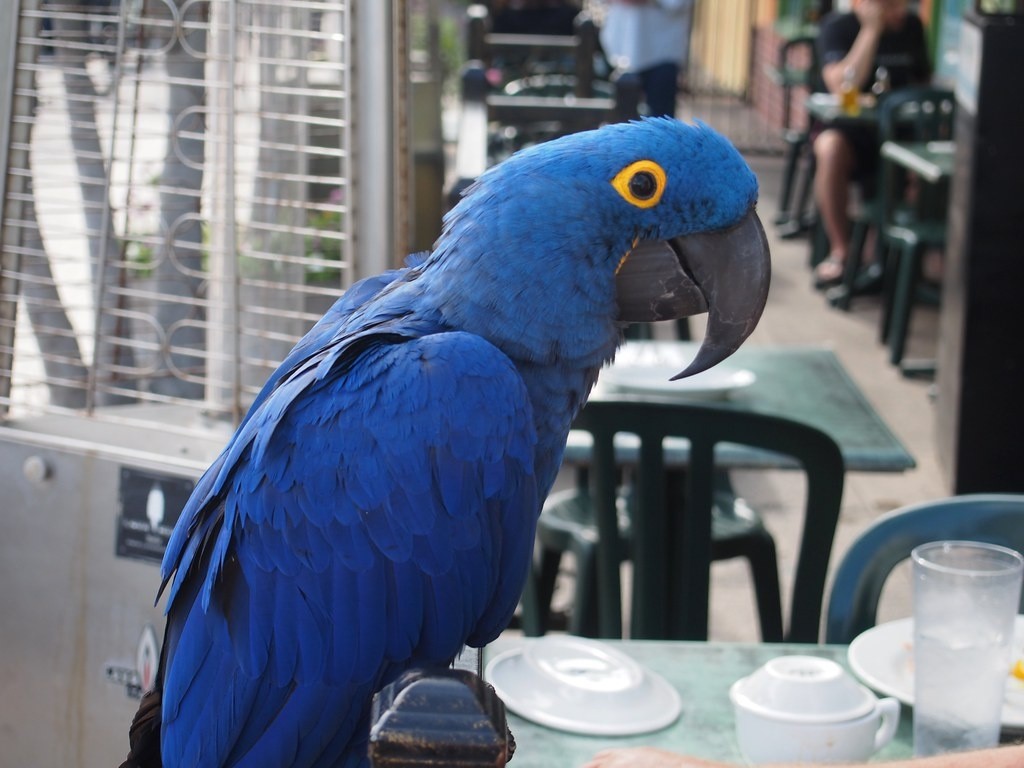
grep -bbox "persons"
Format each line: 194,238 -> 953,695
813,1 -> 949,288
594,0 -> 690,124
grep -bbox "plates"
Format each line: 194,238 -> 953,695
848,617 -> 1024,734
485,635 -> 683,736
594,341 -> 756,401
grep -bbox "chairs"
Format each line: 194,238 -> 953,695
774,38 -> 822,224
823,494 -> 1024,642
879,87 -> 953,364
537,317 -> 785,642
522,400 -> 846,643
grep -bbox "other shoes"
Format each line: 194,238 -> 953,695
814,259 -> 842,287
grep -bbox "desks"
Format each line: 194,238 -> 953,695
448,635 -> 915,768
802,88 -> 953,122
878,137 -> 955,194
564,336 -> 918,528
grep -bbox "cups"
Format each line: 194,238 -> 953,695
730,655 -> 900,762
912,540 -> 1024,759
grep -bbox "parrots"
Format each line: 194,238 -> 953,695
124,113 -> 770,768
